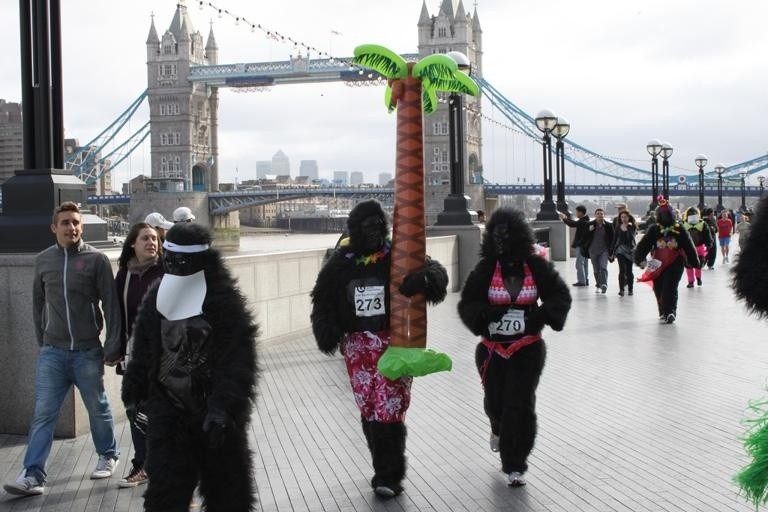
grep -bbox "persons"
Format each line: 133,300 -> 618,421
476,209 -> 487,223
113,206 -> 196,486
556,195 -> 754,325
4,201 -> 121,496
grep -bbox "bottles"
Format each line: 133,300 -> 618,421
615,203 -> 627,208
146,207 -> 195,230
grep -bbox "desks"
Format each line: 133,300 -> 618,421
693,154 -> 766,216
430,50 -> 480,227
658,142 -> 675,202
646,139 -> 663,217
548,117 -> 576,223
534,108 -> 564,222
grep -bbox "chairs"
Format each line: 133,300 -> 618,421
90,455 -> 119,478
4,477 -> 43,495
507,472 -> 526,486
619,290 -> 633,295
601,284 -> 606,293
659,313 -> 675,324
687,279 -> 702,288
491,433 -> 499,452
119,467 -> 149,487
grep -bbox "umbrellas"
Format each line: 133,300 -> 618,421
574,283 -> 585,286
